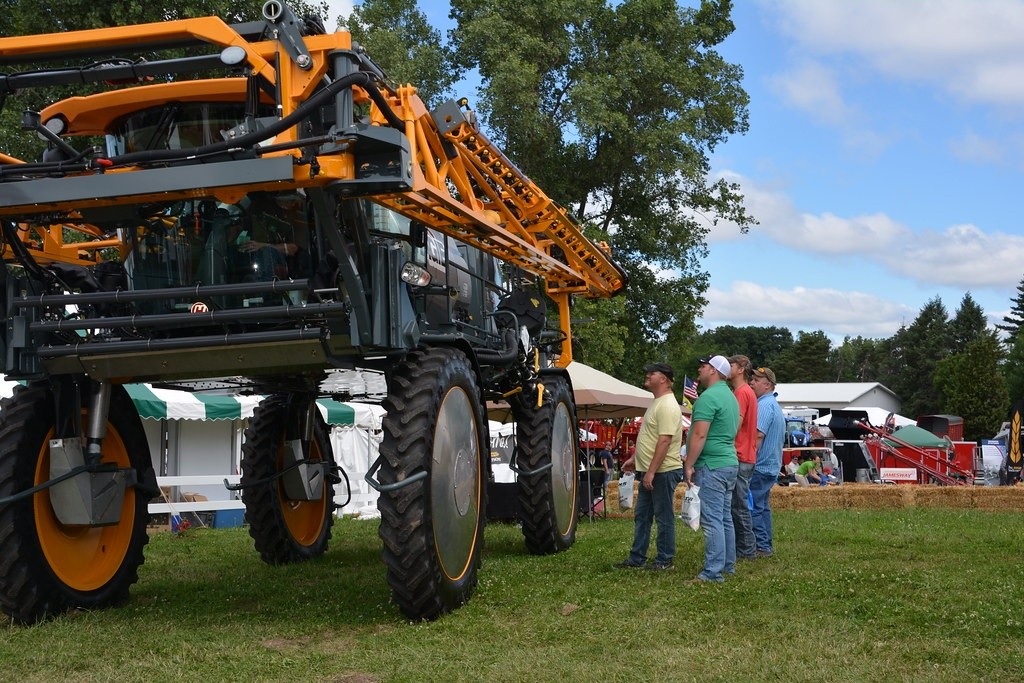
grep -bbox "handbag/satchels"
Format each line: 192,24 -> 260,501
681,482 -> 701,531
618,470 -> 635,513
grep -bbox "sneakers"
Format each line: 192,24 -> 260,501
646,562 -> 674,571
612,558 -> 646,570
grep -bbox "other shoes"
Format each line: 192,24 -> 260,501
685,572 -> 724,586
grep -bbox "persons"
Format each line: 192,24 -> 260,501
599,355 -> 837,583
230,241 -> 314,280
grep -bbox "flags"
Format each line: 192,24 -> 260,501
683,375 -> 698,399
682,395 -> 694,410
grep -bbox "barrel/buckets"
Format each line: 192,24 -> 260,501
215,510 -> 244,528
180,512 -> 215,528
855,469 -> 870,482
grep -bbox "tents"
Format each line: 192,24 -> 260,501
0,373 -> 390,521
879,425 -> 949,487
482,360 -> 655,521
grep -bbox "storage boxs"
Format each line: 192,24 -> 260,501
194,509 -> 247,530
181,492 -> 208,502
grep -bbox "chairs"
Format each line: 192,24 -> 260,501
577,469 -> 607,523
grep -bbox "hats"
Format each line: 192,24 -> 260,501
697,354 -> 731,377
752,367 -> 776,385
606,442 -> 612,446
642,362 -> 673,382
823,468 -> 831,474
725,354 -> 752,374
